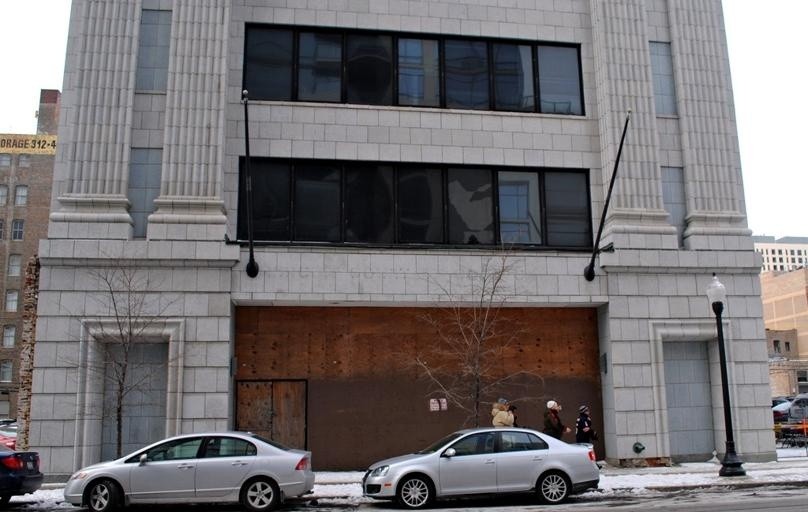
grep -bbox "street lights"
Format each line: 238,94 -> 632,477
705,271 -> 746,477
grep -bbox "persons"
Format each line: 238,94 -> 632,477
508,405 -> 519,427
576,404 -> 602,469
491,398 -> 515,448
542,400 -> 573,440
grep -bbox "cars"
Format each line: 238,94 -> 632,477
362,428 -> 600,508
0,418 -> 43,510
63,431 -> 315,511
772,393 -> 808,446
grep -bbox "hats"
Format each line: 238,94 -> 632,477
578,405 -> 589,414
498,397 -> 507,405
546,400 -> 557,409
